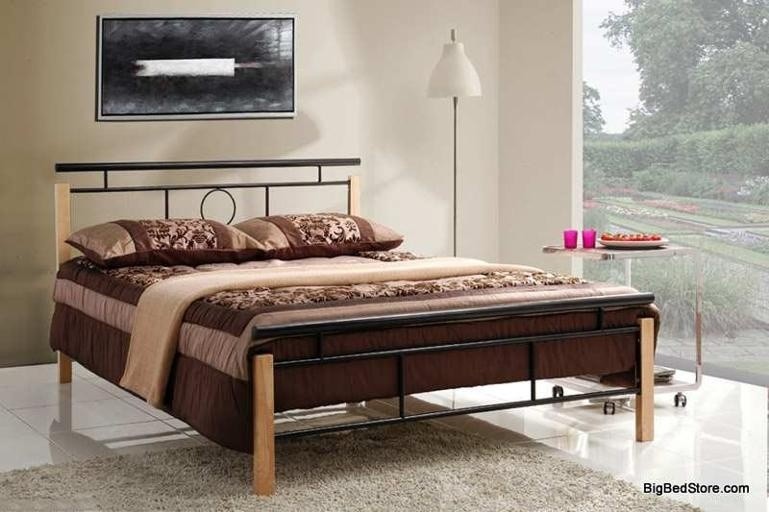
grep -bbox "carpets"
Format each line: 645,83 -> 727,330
1,420 -> 706,512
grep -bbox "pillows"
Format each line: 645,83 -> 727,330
64,219 -> 268,269
232,214 -> 404,260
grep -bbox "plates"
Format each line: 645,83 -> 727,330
596,234 -> 670,248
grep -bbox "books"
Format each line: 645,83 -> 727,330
575,363 -> 676,385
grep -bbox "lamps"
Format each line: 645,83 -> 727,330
426,28 -> 483,257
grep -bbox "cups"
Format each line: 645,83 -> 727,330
563,229 -> 596,249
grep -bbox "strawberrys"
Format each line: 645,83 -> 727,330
602,232 -> 661,240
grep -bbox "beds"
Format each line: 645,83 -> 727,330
53,158 -> 656,496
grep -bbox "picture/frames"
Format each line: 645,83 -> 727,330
95,14 -> 297,121
